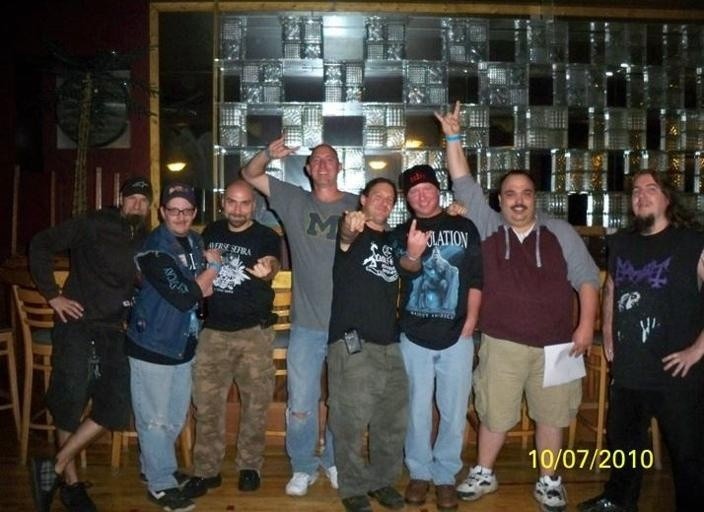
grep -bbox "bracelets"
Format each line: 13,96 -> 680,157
207,262 -> 220,272
402,252 -> 421,262
264,146 -> 272,161
445,134 -> 461,144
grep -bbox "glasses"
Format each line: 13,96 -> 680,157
164,209 -> 195,216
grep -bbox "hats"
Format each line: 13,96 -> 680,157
161,184 -> 197,209
122,178 -> 152,201
404,165 -> 440,197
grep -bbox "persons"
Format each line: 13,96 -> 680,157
433,98 -> 603,511
386,163 -> 483,512
324,178 -> 469,511
24,175 -> 155,511
184,179 -> 282,492
575,166 -> 703,512
121,182 -> 221,511
238,134 -> 390,496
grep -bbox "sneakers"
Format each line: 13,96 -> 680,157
27,454 -> 60,511
286,469 -> 318,496
534,475 -> 566,511
60,480 -> 98,512
139,473 -> 222,512
239,469 -> 260,490
325,466 -> 340,490
457,467 -> 497,501
343,480 -> 457,511
580,496 -> 633,510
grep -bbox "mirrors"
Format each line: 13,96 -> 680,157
148,1 -> 218,233
